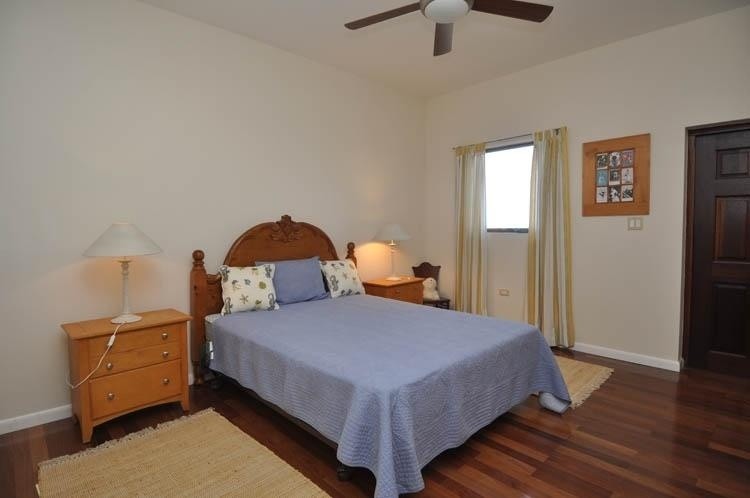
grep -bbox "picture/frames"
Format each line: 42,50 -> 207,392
581,133 -> 651,217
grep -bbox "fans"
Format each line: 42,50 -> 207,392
344,0 -> 554,56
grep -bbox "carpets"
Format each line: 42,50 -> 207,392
35,407 -> 334,498
531,353 -> 614,409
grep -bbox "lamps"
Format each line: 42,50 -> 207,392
373,223 -> 411,281
424,0 -> 469,24
82,223 -> 164,324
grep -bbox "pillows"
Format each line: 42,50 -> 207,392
318,258 -> 370,300
216,263 -> 279,317
255,256 -> 330,304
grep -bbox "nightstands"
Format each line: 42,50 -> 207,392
60,308 -> 193,442
362,276 -> 426,304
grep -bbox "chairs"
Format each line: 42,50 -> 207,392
412,262 -> 450,310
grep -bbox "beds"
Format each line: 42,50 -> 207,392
190,214 -> 540,498
